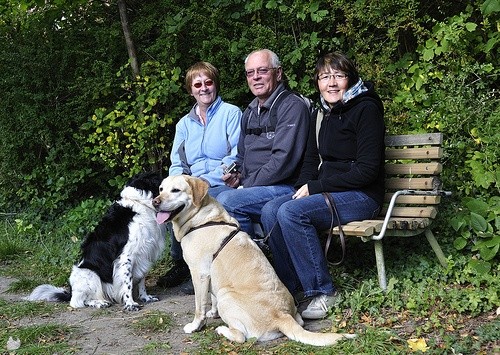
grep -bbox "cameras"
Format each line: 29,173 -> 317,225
223,162 -> 236,175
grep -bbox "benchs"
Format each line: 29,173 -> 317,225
323,133 -> 449,287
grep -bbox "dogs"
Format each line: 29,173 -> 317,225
27,158 -> 170,312
152,174 -> 358,346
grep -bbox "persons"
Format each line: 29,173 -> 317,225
156,61 -> 242,295
261,51 -> 386,319
207,48 -> 315,240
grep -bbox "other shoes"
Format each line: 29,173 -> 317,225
181,280 -> 195,295
157,264 -> 190,288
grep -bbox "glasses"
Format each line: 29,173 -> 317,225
317,70 -> 349,81
245,67 -> 277,77
191,80 -> 214,88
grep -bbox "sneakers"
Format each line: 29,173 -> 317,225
302,291 -> 338,318
